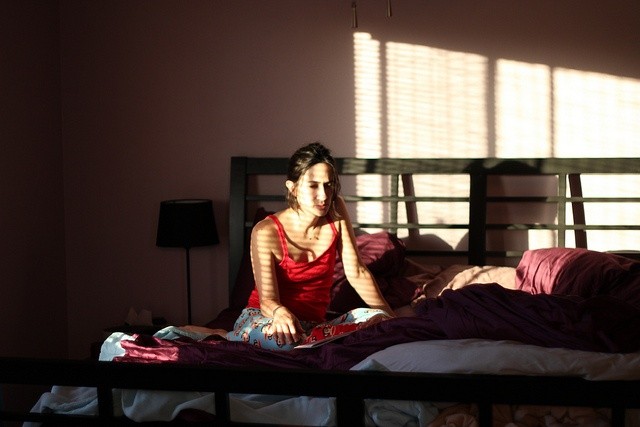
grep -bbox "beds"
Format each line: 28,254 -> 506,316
24,156 -> 639,427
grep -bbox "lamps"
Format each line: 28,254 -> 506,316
154,197 -> 221,325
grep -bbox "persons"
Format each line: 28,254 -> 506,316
177,143 -> 397,353
